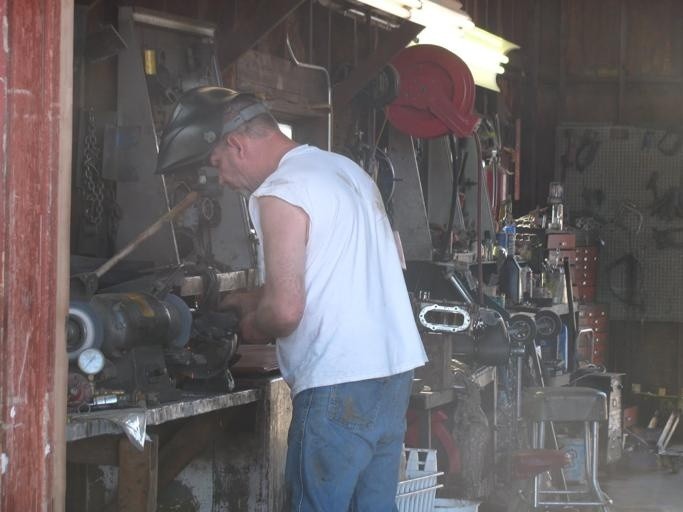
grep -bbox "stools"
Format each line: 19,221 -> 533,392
520,389 -> 613,512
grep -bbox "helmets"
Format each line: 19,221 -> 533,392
154,80 -> 257,176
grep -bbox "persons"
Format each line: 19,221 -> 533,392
202,90 -> 429,512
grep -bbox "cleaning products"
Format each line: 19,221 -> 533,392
496,227 -> 516,258
